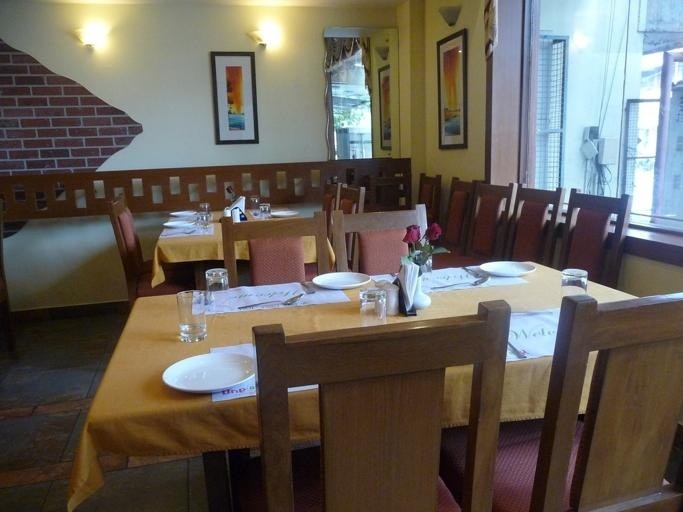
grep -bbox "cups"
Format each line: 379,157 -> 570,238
359,291 -> 388,332
204,269 -> 231,309
196,201 -> 210,233
176,288 -> 207,342
247,195 -> 272,219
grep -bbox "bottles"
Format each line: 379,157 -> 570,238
560,266 -> 588,294
375,279 -> 400,317
397,185 -> 407,210
226,185 -> 242,204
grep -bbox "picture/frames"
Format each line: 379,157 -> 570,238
209,50 -> 259,144
377,64 -> 392,151
435,27 -> 469,150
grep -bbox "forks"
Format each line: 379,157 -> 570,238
432,275 -> 491,291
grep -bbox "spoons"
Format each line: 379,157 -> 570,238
239,293 -> 303,311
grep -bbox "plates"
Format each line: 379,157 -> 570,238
311,271 -> 371,291
480,260 -> 538,276
270,207 -> 299,218
163,208 -> 196,228
160,354 -> 259,392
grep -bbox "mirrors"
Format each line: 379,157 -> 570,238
320,25 -> 402,162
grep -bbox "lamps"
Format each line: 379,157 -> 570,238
245,29 -> 278,49
71,28 -> 104,49
436,4 -> 462,26
